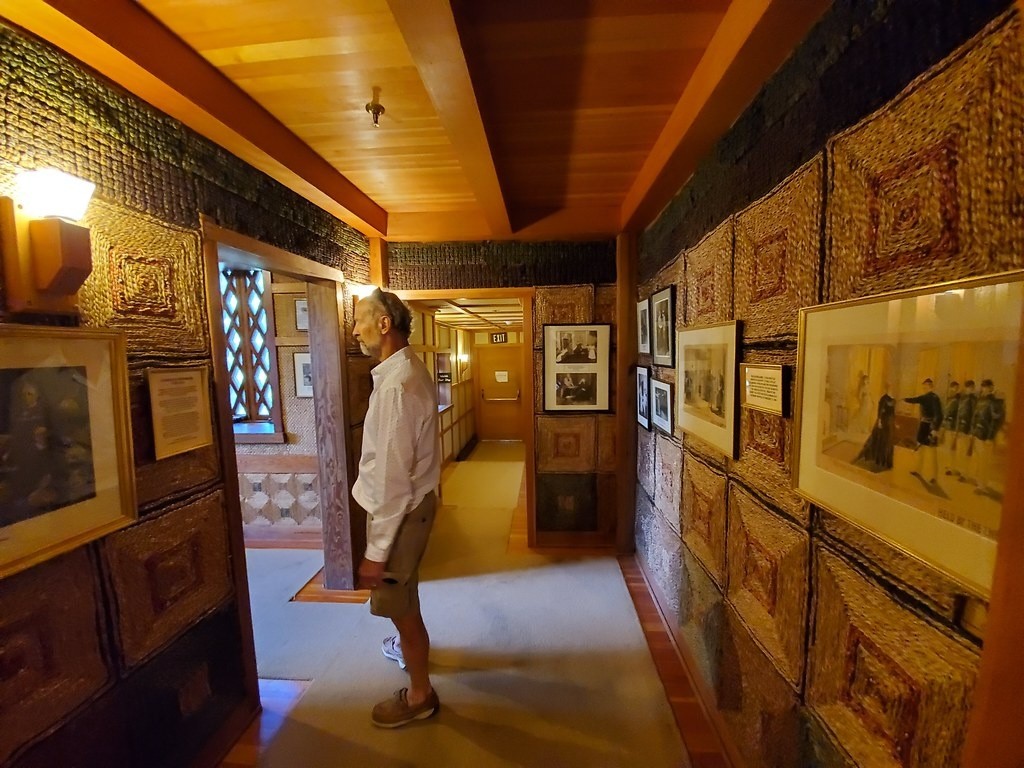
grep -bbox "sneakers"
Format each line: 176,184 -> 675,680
371,688 -> 440,729
381,636 -> 408,672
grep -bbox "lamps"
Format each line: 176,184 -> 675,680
22,167 -> 96,225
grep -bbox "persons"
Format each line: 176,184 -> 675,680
345,288 -> 446,727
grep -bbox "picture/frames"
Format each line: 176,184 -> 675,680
0,323 -> 140,579
144,366 -> 213,460
543,323 -> 610,414
293,352 -> 313,398
294,298 -> 308,331
651,284 -> 674,368
649,378 -> 674,437
739,363 -> 783,417
792,269 -> 1024,602
675,320 -> 739,458
636,298 -> 653,357
636,366 -> 651,431
